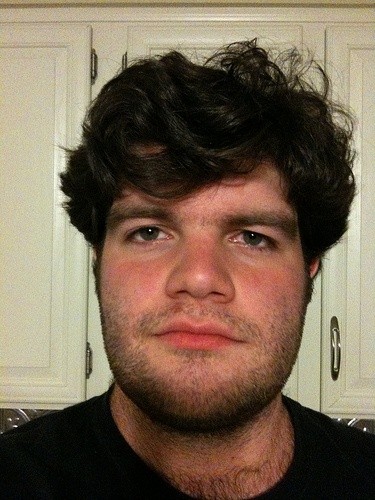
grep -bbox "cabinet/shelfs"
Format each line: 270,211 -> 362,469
0,0 -> 375,419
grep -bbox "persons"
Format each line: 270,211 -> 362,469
0,37 -> 375,500
